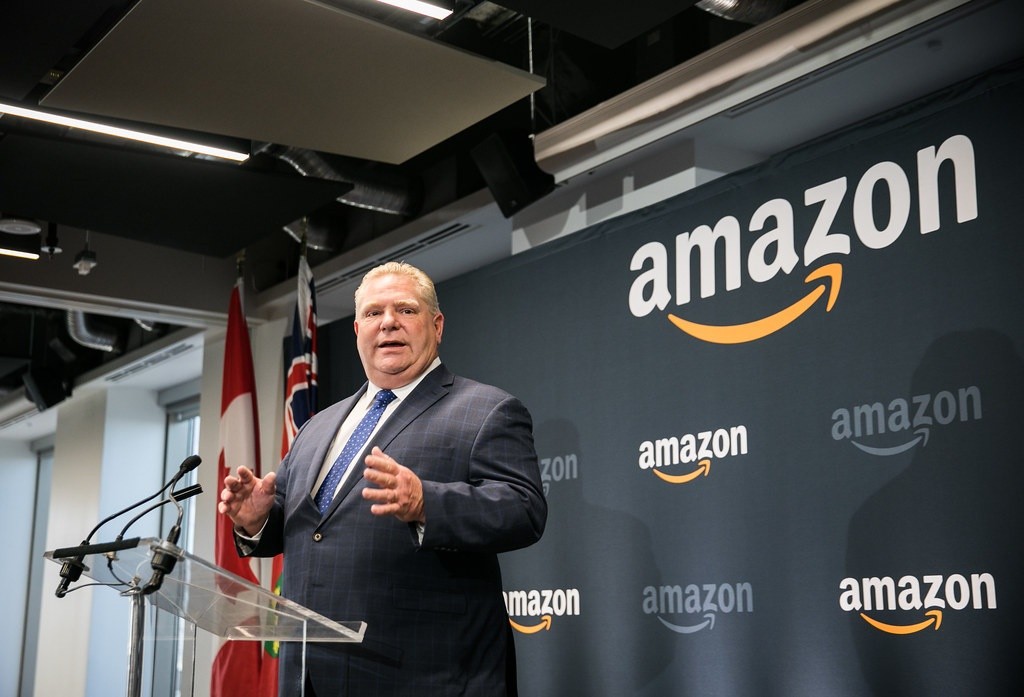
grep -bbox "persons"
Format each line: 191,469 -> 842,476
217,262 -> 548,697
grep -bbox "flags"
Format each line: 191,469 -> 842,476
260,258 -> 317,697
211,278 -> 260,697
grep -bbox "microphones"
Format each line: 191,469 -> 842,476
139,454 -> 202,596
55,456 -> 192,598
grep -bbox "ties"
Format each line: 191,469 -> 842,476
314,388 -> 400,515
